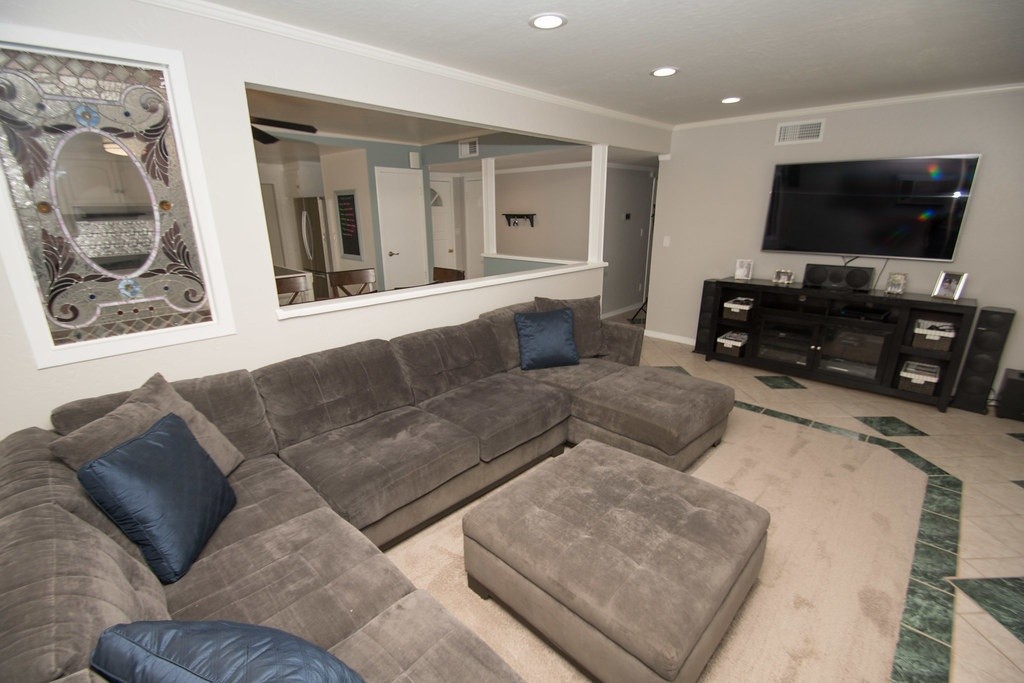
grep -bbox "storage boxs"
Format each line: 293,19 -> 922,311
716,330 -> 748,358
898,360 -> 941,397
723,297 -> 754,322
912,318 -> 956,352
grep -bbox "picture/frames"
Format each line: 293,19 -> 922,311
734,258 -> 753,280
930,270 -> 968,301
884,272 -> 909,294
0,23 -> 239,366
773,268 -> 796,285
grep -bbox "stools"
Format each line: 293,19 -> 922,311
461,438 -> 770,683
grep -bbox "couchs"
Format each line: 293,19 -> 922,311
0,293 -> 738,683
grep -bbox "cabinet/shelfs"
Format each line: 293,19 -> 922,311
693,276 -> 978,414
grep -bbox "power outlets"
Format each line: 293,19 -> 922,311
638,283 -> 641,292
640,227 -> 645,237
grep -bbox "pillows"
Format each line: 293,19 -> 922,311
514,307 -> 580,371
77,411 -> 238,586
46,370 -> 243,480
90,619 -> 367,683
535,295 -> 610,360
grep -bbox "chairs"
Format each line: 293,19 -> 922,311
275,267 -> 379,308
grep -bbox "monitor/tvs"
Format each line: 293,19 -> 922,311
759,154 -> 982,263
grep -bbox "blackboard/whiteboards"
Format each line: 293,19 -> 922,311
333,189 -> 363,261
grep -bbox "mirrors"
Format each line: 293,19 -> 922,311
48,126 -> 162,281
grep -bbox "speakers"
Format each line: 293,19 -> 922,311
802,263 -> 876,292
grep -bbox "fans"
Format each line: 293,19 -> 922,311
248,115 -> 320,144
